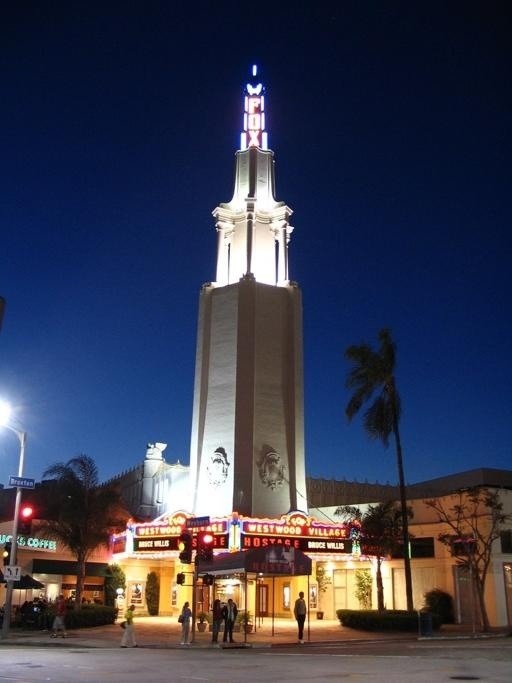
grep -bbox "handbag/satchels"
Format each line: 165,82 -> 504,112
120,621 -> 127,629
179,615 -> 185,623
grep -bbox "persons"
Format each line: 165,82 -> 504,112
294,591 -> 306,644
50,593 -> 68,637
180,602 -> 191,645
1,594 -> 103,612
212,599 -> 224,642
118,605 -> 137,648
221,599 -> 238,643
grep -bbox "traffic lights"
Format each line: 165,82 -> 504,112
17,502 -> 37,535
177,572 -> 185,583
197,530 -> 215,562
203,574 -> 214,585
179,530 -> 193,563
